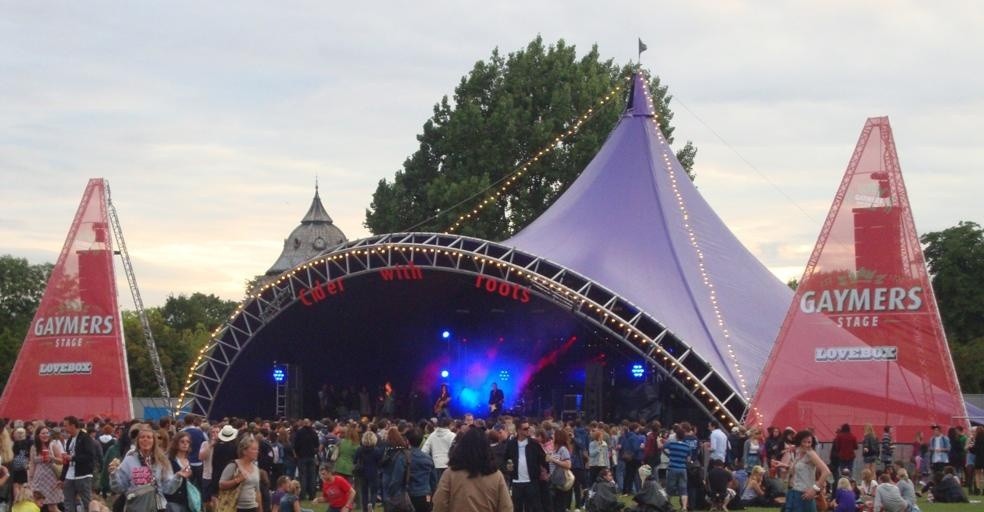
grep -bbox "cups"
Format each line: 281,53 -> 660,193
61,451 -> 69,465
40,449 -> 48,463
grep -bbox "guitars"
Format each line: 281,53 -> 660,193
489,399 -> 504,412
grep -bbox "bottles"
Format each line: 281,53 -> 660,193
506,459 -> 513,471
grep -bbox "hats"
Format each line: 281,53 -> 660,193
217,424 -> 238,442
639,465 -> 652,488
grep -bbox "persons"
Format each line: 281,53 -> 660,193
302,382 -> 373,419
488,382 -> 504,415
433,384 -> 451,417
380,382 -> 396,417
2,414 -> 982,512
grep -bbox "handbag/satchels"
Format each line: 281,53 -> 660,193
388,494 -> 412,511
123,485 -> 157,512
549,467 -> 574,491
186,478 -> 202,512
215,461 -> 244,511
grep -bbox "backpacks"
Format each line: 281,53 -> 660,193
324,436 -> 339,461
